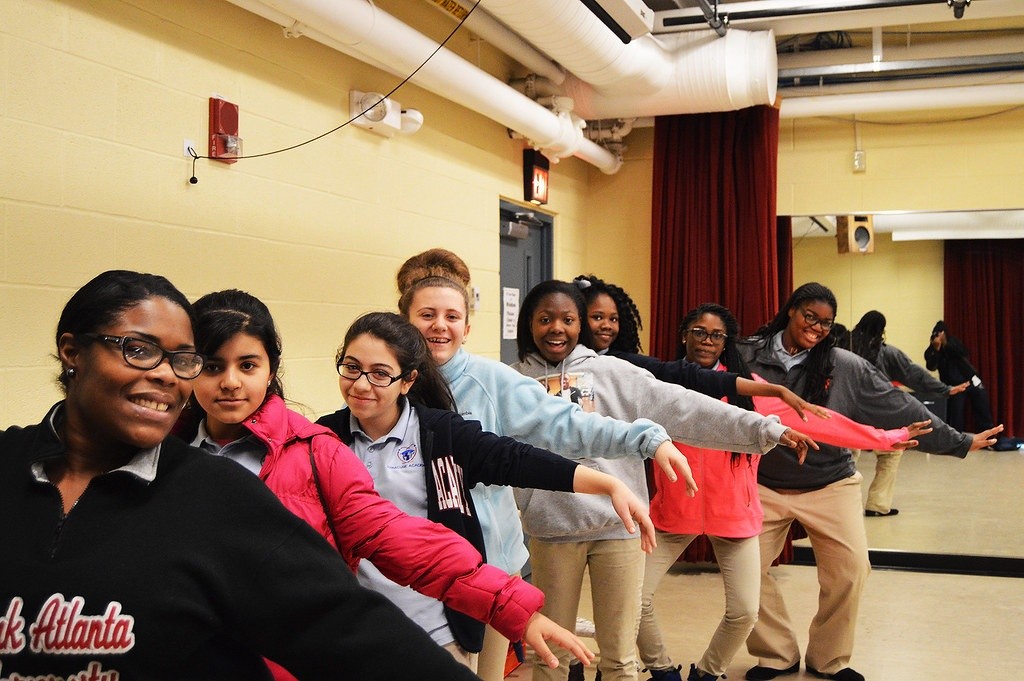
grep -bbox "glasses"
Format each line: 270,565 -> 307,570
798,305 -> 835,331
685,327 -> 728,345
74,331 -> 208,380
336,356 -> 410,388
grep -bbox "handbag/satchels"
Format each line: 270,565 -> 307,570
994,439 -> 1022,451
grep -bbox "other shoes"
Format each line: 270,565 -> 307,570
805,662 -> 864,681
746,662 -> 800,681
642,665 -> 682,681
688,663 -> 718,681
865,506 -> 898,517
568,662 -> 585,681
574,616 -> 597,638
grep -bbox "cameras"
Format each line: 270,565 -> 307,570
931,331 -> 939,340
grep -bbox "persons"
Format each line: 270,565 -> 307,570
504,280 -> 819,681
636,302 -> 932,681
734,285 -> 1005,681
397,248 -> 698,680
840,309 -> 972,516
568,274 -> 833,681
924,320 -> 989,451
0,269 -> 485,681
165,288 -> 597,681
312,312 -> 658,678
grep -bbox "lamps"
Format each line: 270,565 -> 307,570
349,89 -> 424,138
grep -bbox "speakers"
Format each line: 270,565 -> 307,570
836,214 -> 874,254
